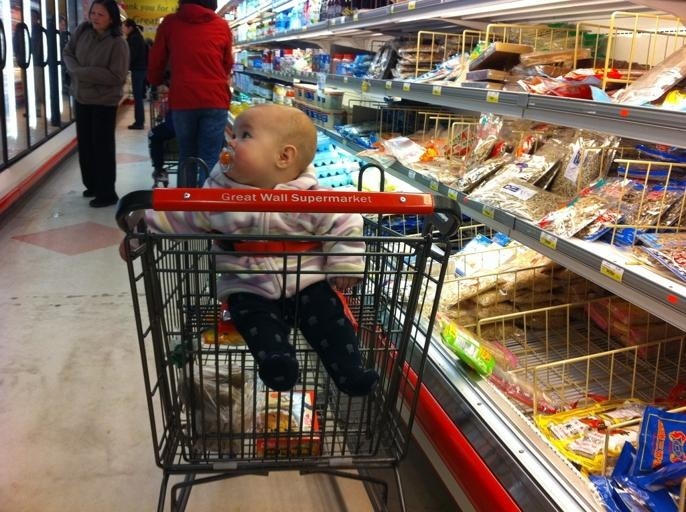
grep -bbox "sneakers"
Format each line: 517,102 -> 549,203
84,189 -> 97,197
89,191 -> 120,209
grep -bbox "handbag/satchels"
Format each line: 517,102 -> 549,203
63,25 -> 89,94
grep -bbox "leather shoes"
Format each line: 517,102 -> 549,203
128,124 -> 145,128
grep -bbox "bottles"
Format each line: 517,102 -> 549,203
253,79 -> 295,106
331,55 -> 354,78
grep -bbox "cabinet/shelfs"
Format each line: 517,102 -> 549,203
231,0 -> 685,512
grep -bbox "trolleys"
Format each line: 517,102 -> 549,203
118,165 -> 461,512
148,78 -> 203,188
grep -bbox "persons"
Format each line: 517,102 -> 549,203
146,0 -> 234,189
126,102 -> 379,400
61,0 -> 130,207
122,19 -> 147,130
